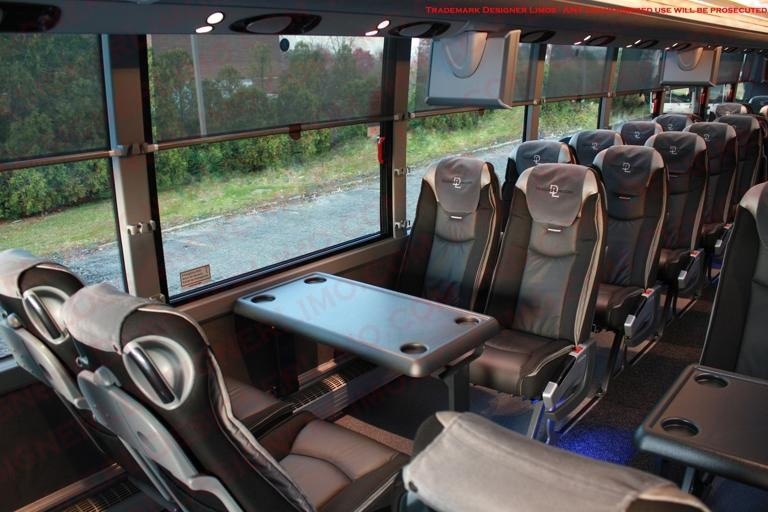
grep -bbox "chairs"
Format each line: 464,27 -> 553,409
62,283 -> 411,511
0,248 -> 295,493
398,411 -> 707,511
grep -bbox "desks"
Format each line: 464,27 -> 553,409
237,271 -> 499,412
635,362 -> 768,511
